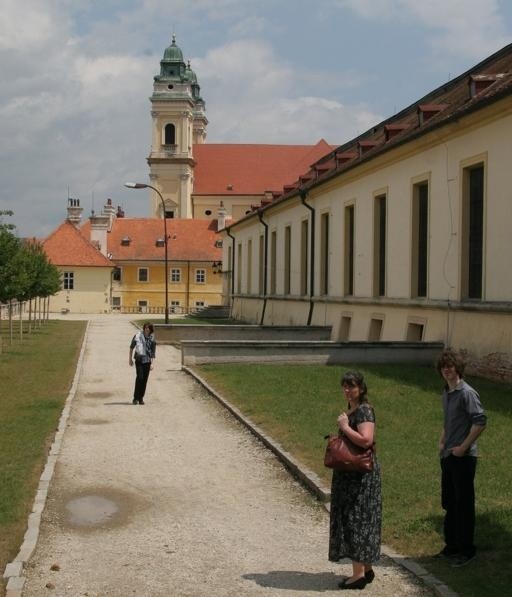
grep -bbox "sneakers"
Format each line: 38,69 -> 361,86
133,398 -> 144,406
431,545 -> 478,570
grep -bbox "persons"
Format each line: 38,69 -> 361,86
128,323 -> 157,405
328,368 -> 383,591
430,349 -> 488,568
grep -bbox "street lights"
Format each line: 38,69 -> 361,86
123,183 -> 169,324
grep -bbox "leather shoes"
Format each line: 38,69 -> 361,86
338,570 -> 376,591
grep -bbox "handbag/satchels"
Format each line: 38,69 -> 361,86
323,433 -> 375,473
